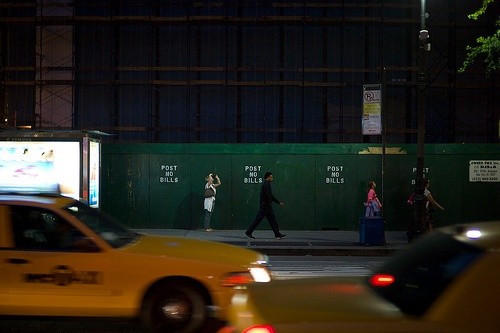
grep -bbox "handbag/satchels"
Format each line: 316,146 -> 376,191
203,188 -> 214,198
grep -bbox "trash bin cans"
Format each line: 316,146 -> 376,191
359,217 -> 384,245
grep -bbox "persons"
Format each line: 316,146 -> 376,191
244,172 -> 286,240
41,152 -> 48,161
367,180 -> 380,217
46,150 -> 55,159
23,148 -> 30,162
424,178 -> 445,233
203,172 -> 221,232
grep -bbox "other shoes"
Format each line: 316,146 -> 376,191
275,233 -> 286,239
201,228 -> 214,232
244,231 -> 255,240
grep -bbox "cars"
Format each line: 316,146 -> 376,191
218,221 -> 499,333
0,187 -> 271,333
